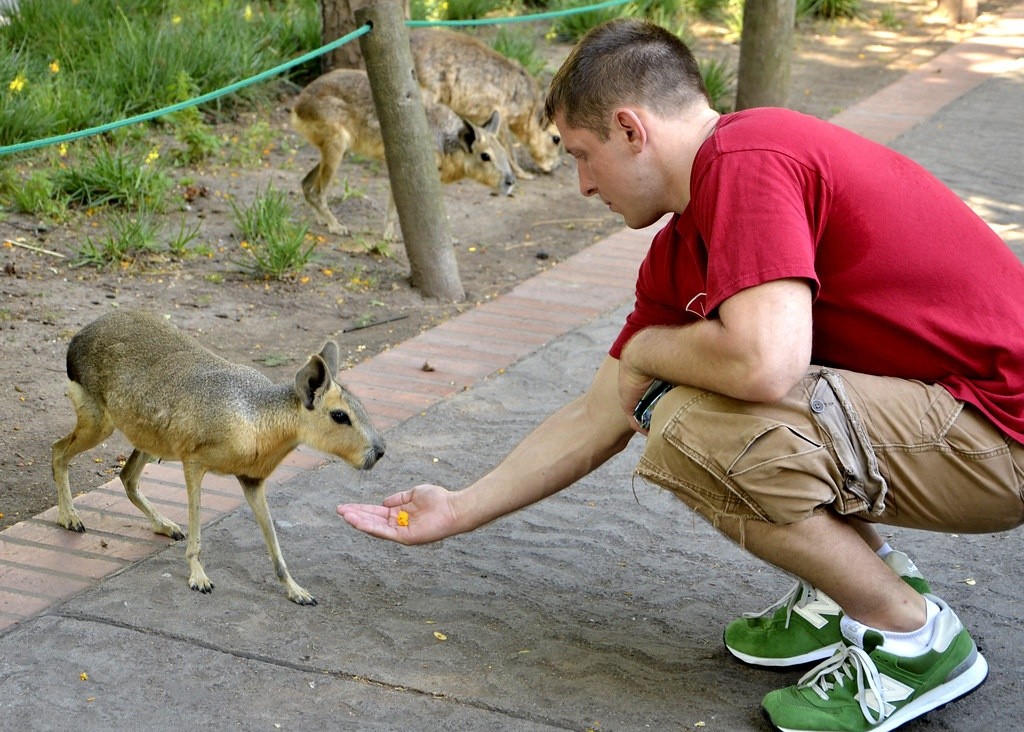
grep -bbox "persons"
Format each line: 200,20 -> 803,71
333,16 -> 1023,732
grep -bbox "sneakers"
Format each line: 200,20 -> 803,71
761,592 -> 989,732
723,549 -> 933,670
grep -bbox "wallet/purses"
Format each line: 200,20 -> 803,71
633,379 -> 674,429
